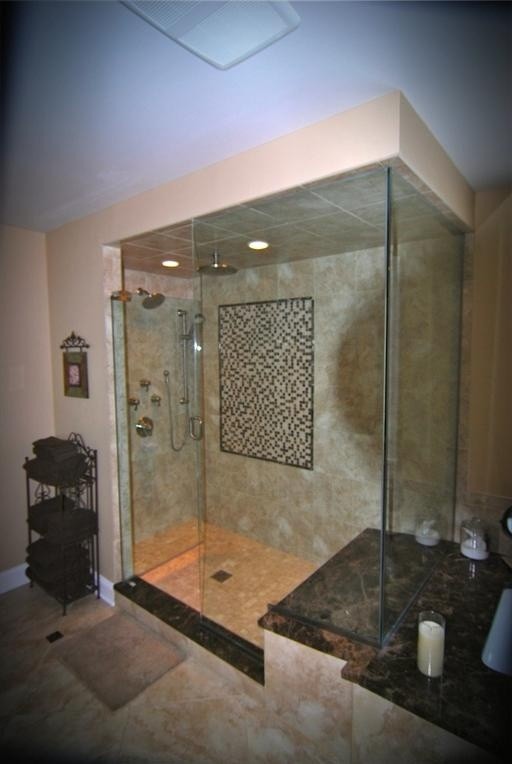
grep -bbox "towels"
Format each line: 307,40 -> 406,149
28,436 -> 96,598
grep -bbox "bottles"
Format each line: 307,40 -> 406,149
460,518 -> 491,561
415,513 -> 443,547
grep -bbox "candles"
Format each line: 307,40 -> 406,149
416,620 -> 446,677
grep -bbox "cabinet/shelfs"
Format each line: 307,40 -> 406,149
21,431 -> 101,615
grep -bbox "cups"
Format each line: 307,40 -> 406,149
418,611 -> 447,678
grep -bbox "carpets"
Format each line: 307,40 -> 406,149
47,609 -> 187,713
